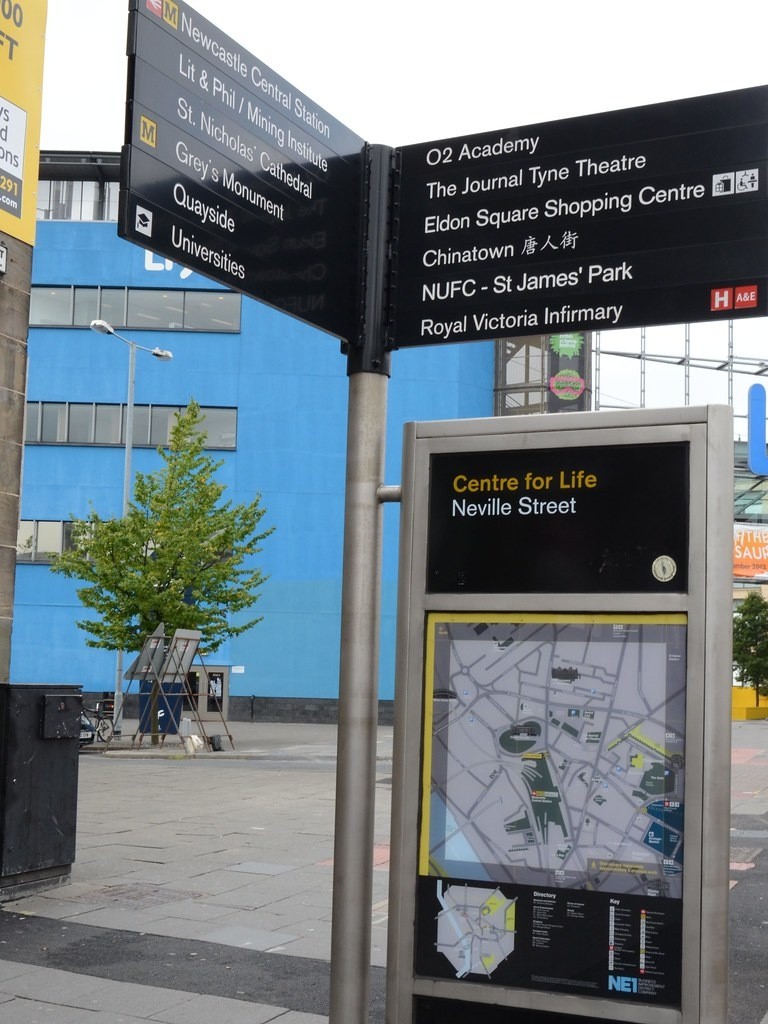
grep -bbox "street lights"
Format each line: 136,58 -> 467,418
88,318 -> 173,748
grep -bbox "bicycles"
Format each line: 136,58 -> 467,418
80,705 -> 114,743
